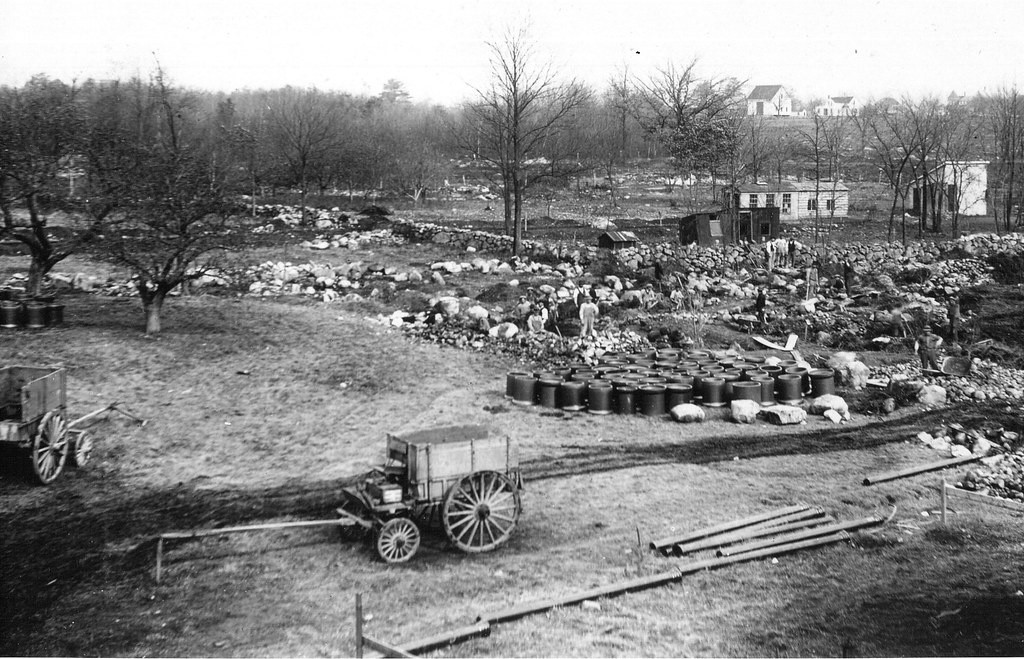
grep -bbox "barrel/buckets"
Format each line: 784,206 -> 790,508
0,289 -> 66,328
505,347 -> 836,416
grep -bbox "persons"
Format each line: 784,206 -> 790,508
805,261 -> 820,300
766,235 -> 795,271
638,284 -> 658,310
670,285 -> 683,312
891,304 -> 908,338
574,281 -> 599,338
914,326 -> 943,376
844,254 -> 855,297
947,296 -> 962,343
754,288 -> 766,322
516,284 -> 558,337
830,274 -> 845,299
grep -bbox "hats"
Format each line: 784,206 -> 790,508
646,284 -> 653,287
922,326 -> 933,331
532,307 -> 540,312
770,235 -> 776,239
790,236 -> 794,240
584,296 -> 593,299
579,281 -> 585,285
